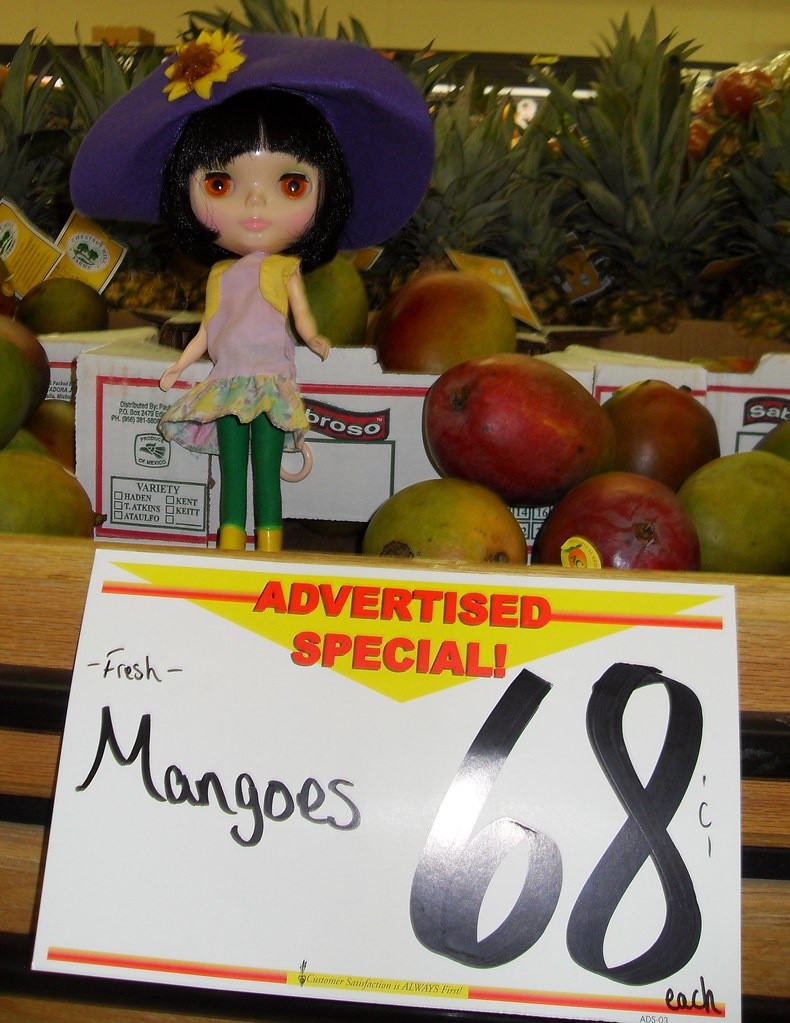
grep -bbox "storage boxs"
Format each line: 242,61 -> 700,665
564,346 -> 790,456
74,337 -> 594,565
38,328 -> 160,403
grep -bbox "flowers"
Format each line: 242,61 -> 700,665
162,30 -> 247,101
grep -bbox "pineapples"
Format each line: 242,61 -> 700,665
0,0 -> 790,357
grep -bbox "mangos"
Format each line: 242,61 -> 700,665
0,260 -> 110,538
295,258 -> 790,579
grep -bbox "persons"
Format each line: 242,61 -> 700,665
71,28 -> 435,554
